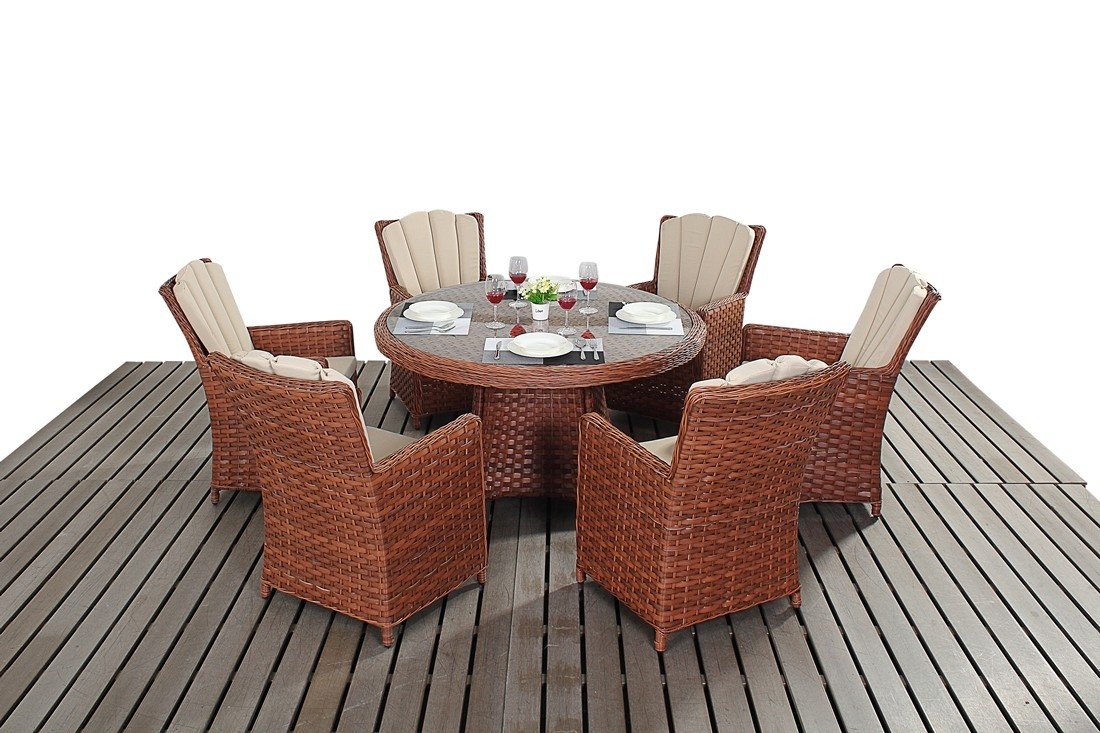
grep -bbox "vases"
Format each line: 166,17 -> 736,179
531,302 -> 549,320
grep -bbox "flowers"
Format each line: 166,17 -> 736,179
519,276 -> 559,303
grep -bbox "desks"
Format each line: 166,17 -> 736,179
373,280 -> 708,501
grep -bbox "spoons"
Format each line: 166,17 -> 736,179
405,321 -> 455,329
576,336 -> 588,360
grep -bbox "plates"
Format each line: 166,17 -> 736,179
402,301 -> 465,322
525,276 -> 577,293
615,302 -> 677,325
507,332 -> 574,358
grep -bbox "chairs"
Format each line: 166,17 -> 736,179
604,213 -> 766,423
575,354 -> 850,651
159,256 -> 363,503
207,349 -> 492,649
741,263 -> 942,516
374,209 -> 487,430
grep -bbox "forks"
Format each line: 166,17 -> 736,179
404,326 -> 455,333
590,338 -> 600,361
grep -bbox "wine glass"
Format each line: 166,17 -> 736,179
485,274 -> 505,329
556,279 -> 578,335
579,262 -> 598,315
509,307 -> 527,337
580,313 -> 596,339
509,256 -> 528,308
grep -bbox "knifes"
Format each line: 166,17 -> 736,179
619,326 -> 673,330
494,341 -> 502,359
